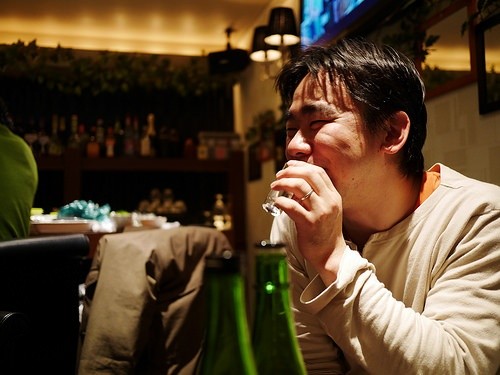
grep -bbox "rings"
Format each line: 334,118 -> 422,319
300,189 -> 314,202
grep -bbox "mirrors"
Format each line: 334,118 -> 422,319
417,0 -> 478,103
473,13 -> 500,115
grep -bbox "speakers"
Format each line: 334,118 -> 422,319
207,49 -> 251,75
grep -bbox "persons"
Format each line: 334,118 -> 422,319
270,36 -> 500,375
0,98 -> 38,242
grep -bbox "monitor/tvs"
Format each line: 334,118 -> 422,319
299,0 -> 375,56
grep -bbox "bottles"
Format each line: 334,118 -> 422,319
149,188 -> 174,214
214,192 -> 230,227
251,240 -> 309,375
198,256 -> 257,375
15,114 -> 160,163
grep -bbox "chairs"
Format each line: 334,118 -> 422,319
0,231 -> 91,375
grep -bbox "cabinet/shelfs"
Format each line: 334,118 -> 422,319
34,147 -> 245,249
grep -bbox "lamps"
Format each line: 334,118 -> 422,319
248,25 -> 280,79
264,7 -> 300,63
